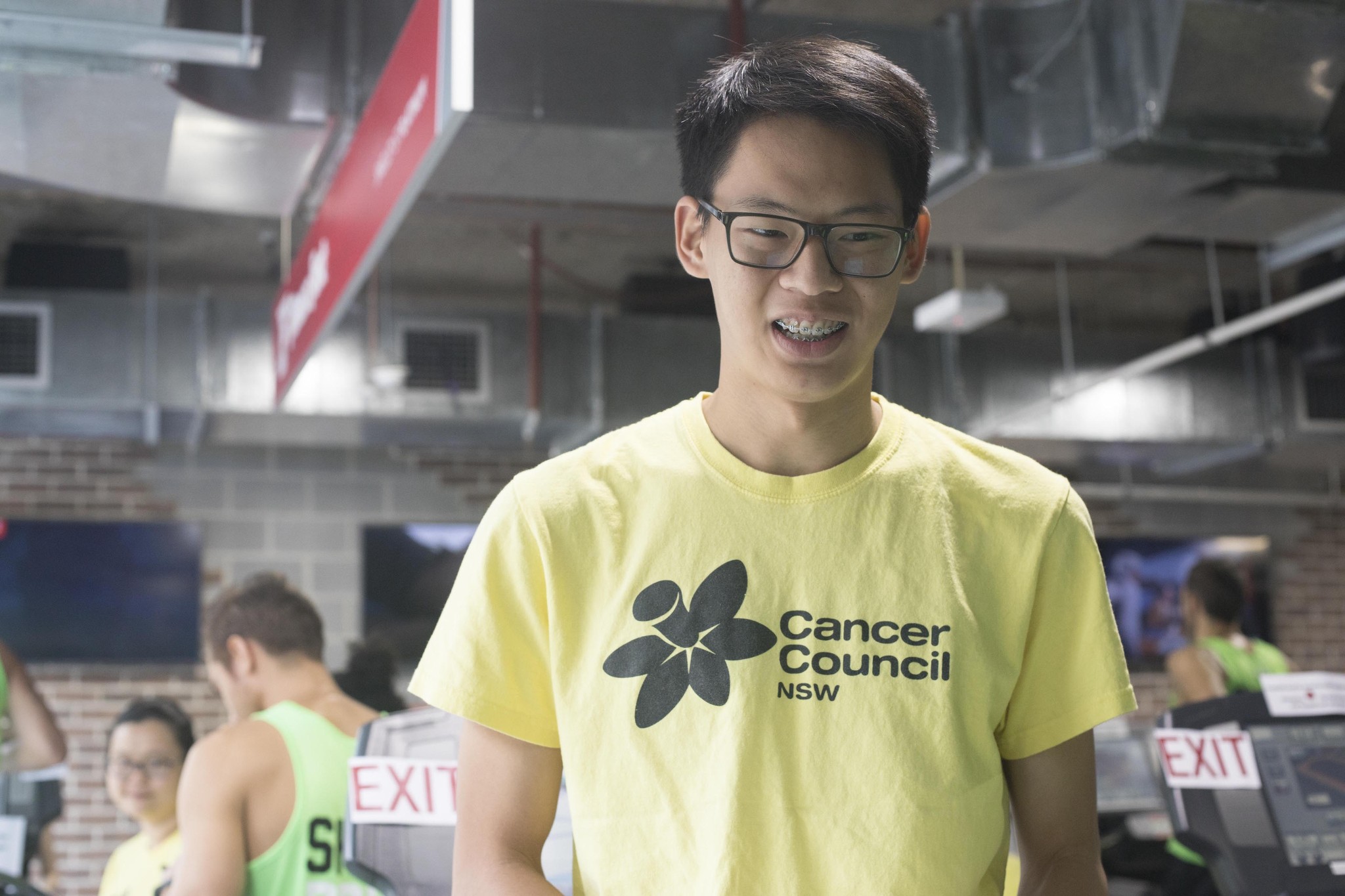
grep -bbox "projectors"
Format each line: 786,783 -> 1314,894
912,289 -> 1007,334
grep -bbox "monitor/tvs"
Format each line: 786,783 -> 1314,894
359,522 -> 484,672
1248,720 -> 1345,866
0,518 -> 205,667
1096,532 -> 1276,670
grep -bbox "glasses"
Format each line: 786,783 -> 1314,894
699,198 -> 918,278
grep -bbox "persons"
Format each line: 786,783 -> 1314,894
168,571 -> 387,896
409,39 -> 1141,896
1166,560 -> 1302,896
19,760 -> 68,893
99,695 -> 200,896
0,644 -> 67,778
